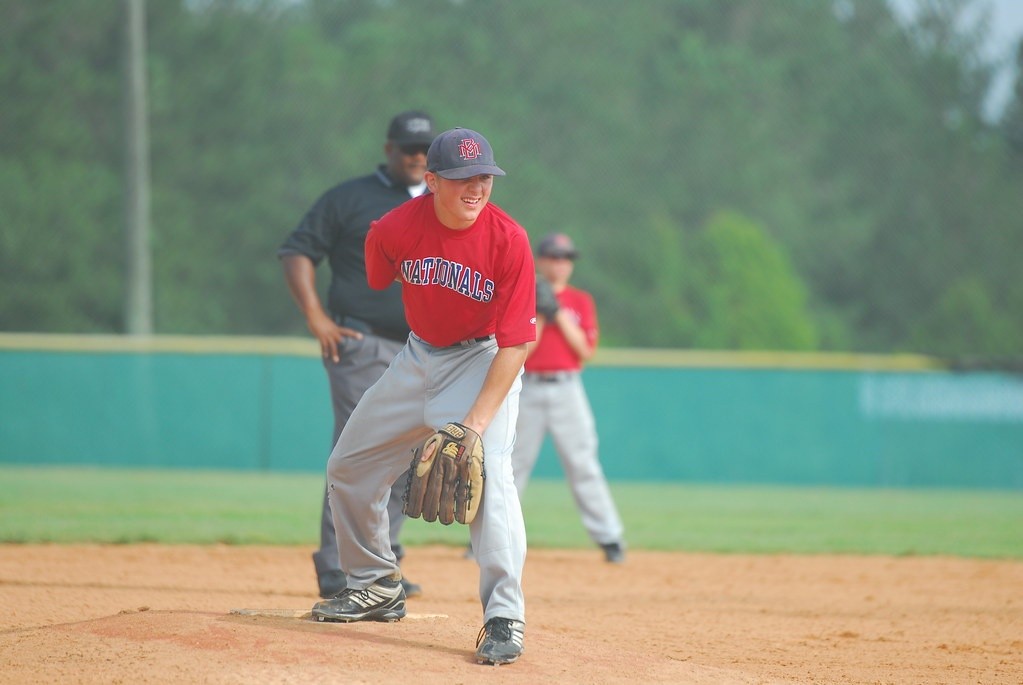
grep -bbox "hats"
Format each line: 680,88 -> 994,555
386,112 -> 436,145
425,126 -> 506,181
536,233 -> 579,261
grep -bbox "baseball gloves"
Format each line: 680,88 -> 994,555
401,420 -> 485,524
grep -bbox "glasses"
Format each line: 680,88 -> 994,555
398,145 -> 430,156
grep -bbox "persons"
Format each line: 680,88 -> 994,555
467,231 -> 626,565
310,125 -> 529,666
276,110 -> 434,600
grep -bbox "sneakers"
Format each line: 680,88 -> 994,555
399,576 -> 422,599
600,539 -> 625,564
463,543 -> 475,560
475,617 -> 526,666
311,576 -> 407,624
